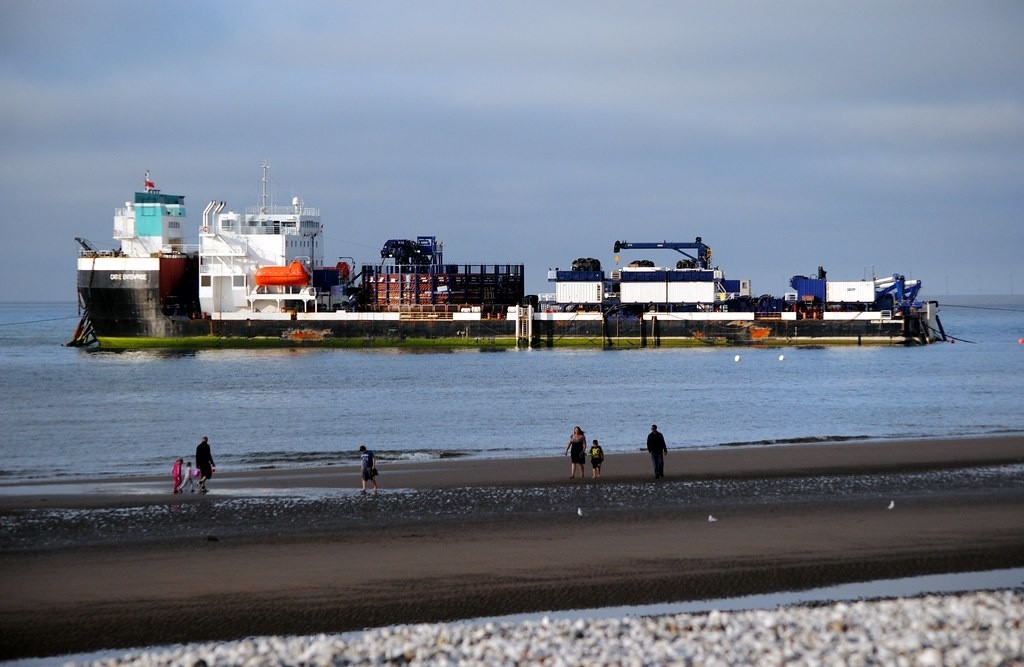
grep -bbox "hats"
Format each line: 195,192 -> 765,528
650,425 -> 657,429
176,458 -> 183,461
358,446 -> 366,452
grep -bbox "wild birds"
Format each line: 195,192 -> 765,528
708,514 -> 718,523
887,501 -> 896,510
577,507 -> 585,516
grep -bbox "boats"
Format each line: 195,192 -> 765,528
68,158 -> 977,348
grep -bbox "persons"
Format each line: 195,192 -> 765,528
196,436 -> 215,489
171,458 -> 183,494
565,427 -> 586,479
358,446 -> 378,492
176,461 -> 195,493
588,440 -> 604,479
647,425 -> 667,479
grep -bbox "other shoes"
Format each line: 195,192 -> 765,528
592,475 -> 596,479
196,481 -> 205,489
597,475 -> 601,478
174,490 -> 183,494
199,489 -> 209,493
579,477 -> 584,481
189,490 -> 195,493
176,488 -> 182,493
569,476 -> 574,479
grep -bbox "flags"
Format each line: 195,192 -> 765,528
146,182 -> 154,187
